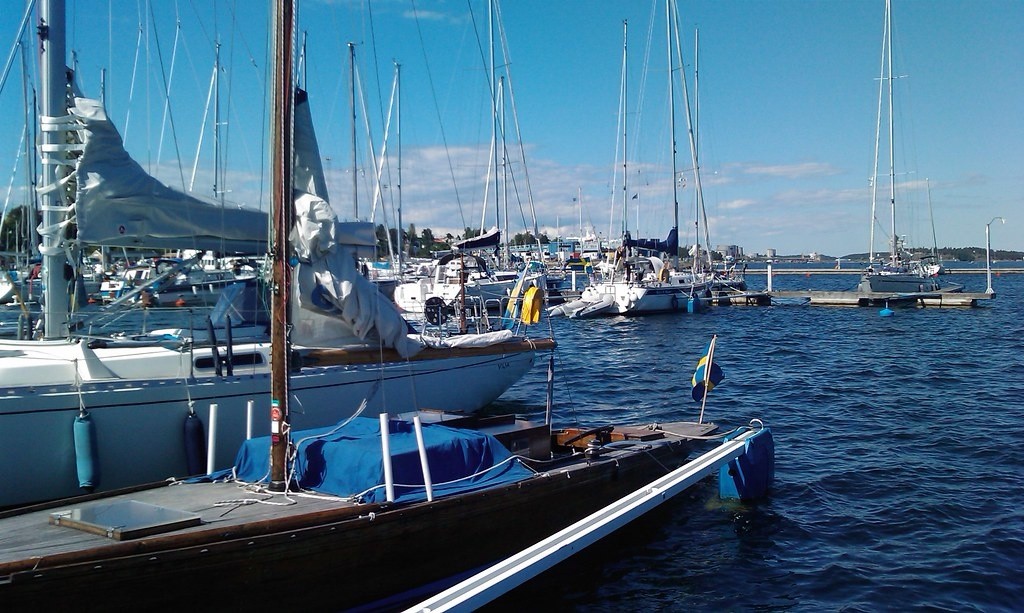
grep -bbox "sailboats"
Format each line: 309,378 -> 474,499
0,1 -> 768,613
868,1 -> 945,293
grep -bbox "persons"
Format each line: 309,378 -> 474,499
659,261 -> 669,284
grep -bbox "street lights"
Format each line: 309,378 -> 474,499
984,216 -> 1006,294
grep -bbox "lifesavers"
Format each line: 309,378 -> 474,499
521,286 -> 542,326
659,268 -> 670,283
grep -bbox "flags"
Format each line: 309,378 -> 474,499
500,259 -> 529,331
691,337 -> 725,403
